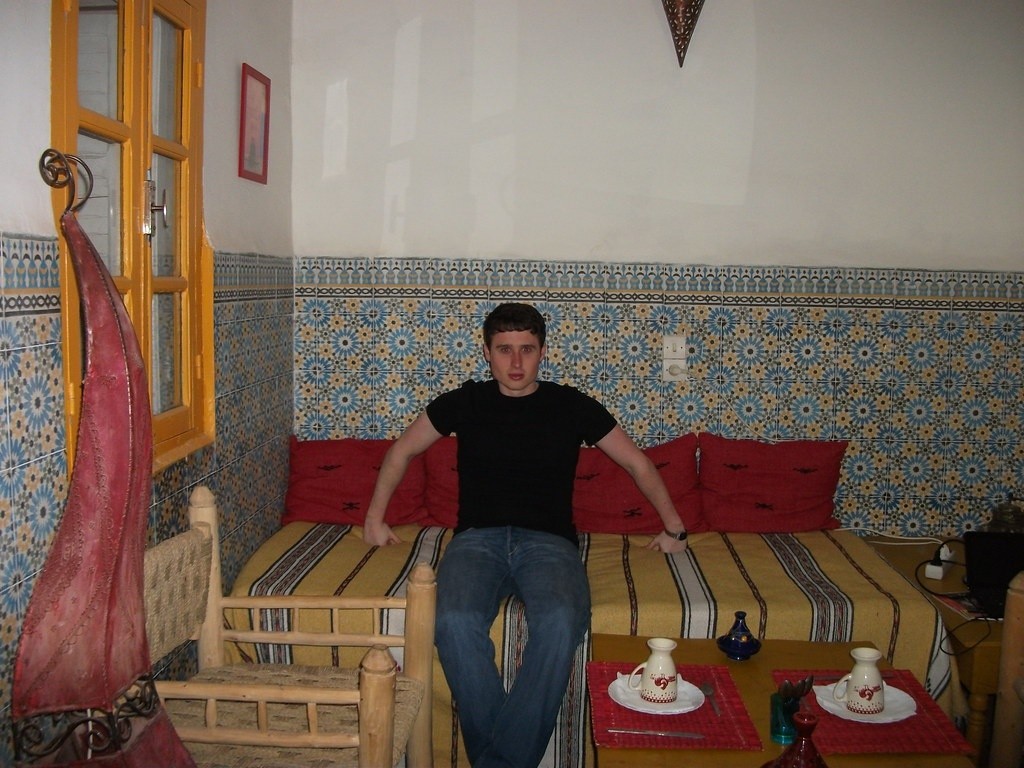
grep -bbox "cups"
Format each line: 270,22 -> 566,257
769,692 -> 800,746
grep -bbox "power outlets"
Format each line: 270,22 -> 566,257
663,359 -> 687,382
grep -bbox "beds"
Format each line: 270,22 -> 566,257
220,520 -> 968,768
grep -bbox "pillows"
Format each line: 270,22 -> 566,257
572,433 -> 710,535
417,436 -> 458,528
698,432 -> 847,531
281,435 -> 428,527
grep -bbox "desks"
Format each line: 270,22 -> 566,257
864,535 -> 1004,768
584,632 -> 975,768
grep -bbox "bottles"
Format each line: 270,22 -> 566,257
832,648 -> 885,715
629,638 -> 677,702
757,711 -> 831,768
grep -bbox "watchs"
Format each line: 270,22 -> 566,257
665,528 -> 688,541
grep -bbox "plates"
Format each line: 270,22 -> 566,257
816,682 -> 916,723
608,675 -> 706,715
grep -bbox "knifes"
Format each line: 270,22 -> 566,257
815,675 -> 896,680
607,728 -> 705,738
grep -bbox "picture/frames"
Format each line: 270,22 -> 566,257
238,62 -> 272,185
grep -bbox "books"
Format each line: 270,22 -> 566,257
932,594 -> 1003,621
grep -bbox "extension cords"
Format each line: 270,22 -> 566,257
926,550 -> 955,579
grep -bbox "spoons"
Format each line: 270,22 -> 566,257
700,682 -> 721,716
777,674 -> 813,706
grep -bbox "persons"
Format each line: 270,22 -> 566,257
363,303 -> 689,768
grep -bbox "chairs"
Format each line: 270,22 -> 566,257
143,485 -> 437,768
